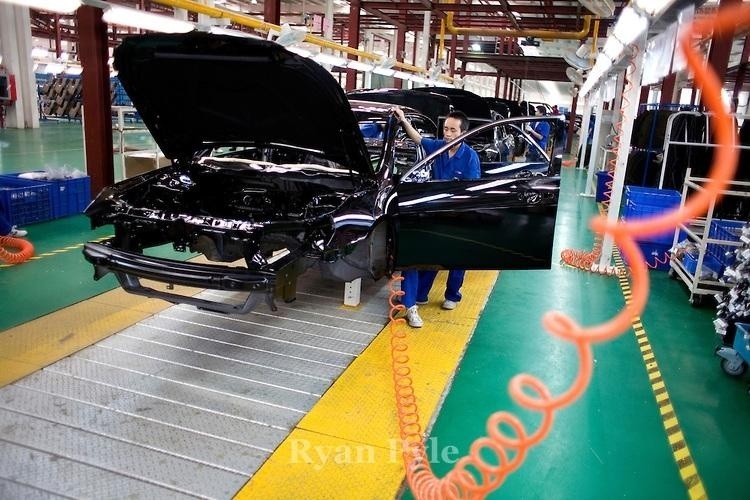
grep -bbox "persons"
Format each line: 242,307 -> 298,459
0,212 -> 28,237
361,105 -> 483,329
548,104 -> 558,117
524,104 -> 550,162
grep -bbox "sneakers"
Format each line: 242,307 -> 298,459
442,300 -> 457,309
406,307 -> 423,327
397,295 -> 428,304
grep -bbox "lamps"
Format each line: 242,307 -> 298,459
100,6 -> 456,93
561,1 -> 674,101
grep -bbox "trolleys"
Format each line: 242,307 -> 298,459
716,322 -> 750,376
668,168 -> 750,307
